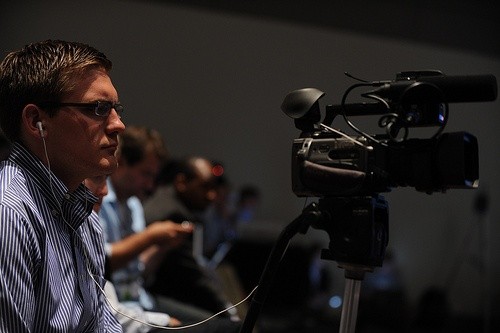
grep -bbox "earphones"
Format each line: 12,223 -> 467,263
36,121 -> 42,131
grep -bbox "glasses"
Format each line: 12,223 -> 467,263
57,99 -> 127,118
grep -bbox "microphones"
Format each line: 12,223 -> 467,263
374,75 -> 498,102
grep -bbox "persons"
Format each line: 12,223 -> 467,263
0,39 -> 127,333
80,124 -> 264,333
